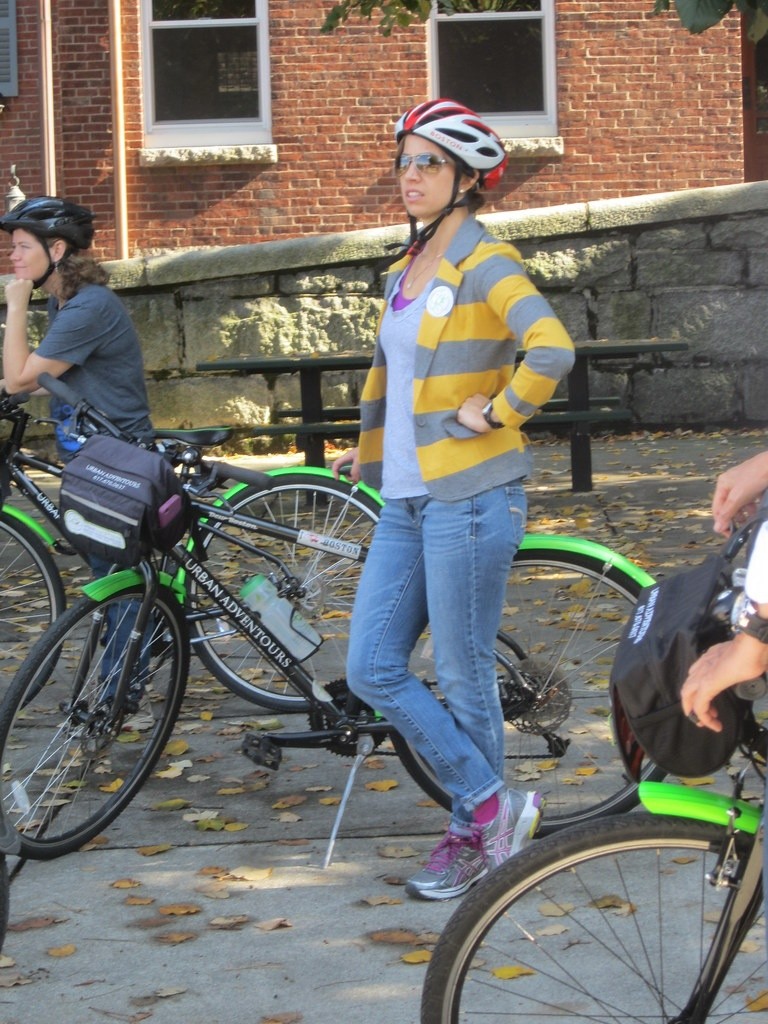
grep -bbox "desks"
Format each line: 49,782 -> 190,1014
194,335 -> 689,493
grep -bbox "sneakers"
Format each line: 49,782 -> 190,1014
404,817 -> 488,899
62,691 -> 154,739
470,788 -> 547,871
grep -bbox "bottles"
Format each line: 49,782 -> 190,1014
238,573 -> 323,660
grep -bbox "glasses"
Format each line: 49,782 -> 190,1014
396,153 -> 455,178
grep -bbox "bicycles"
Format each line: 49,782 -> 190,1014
1,366 -> 679,859
419,483 -> 768,1021
0,375 -> 388,721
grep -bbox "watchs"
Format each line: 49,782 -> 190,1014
481,402 -> 504,430
730,591 -> 768,645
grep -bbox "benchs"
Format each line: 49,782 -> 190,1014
254,392 -> 629,438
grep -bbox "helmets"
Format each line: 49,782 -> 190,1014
395,98 -> 508,190
55,419 -> 93,465
0,196 -> 97,248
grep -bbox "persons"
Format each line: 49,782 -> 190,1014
0,196 -> 156,738
332,99 -> 576,900
680,450 -> 768,734
